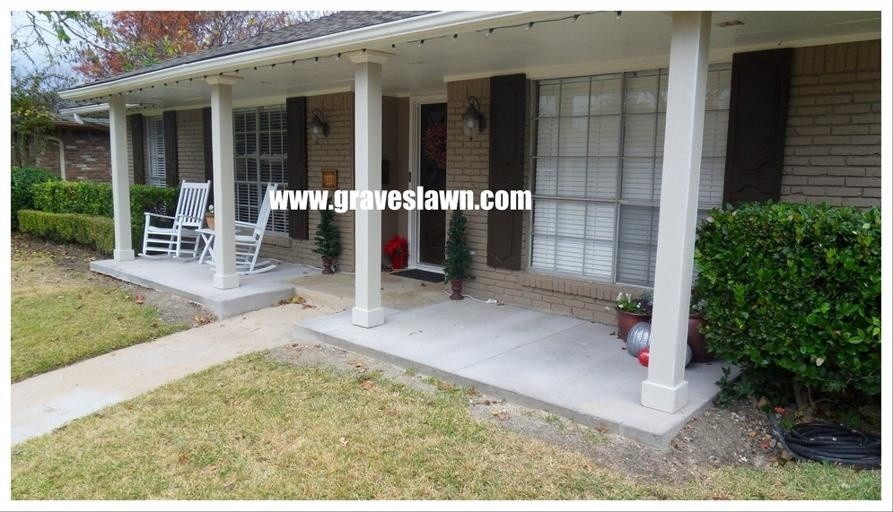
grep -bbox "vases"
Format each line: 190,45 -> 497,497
205,213 -> 214,229
688,314 -> 713,363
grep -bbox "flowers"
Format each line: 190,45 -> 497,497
206,204 -> 213,214
616,290 -> 646,314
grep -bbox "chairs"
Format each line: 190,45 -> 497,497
233,182 -> 278,277
136,178 -> 211,262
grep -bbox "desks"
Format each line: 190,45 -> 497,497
195,227 -> 242,263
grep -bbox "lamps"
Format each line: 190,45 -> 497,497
460,95 -> 487,144
308,107 -> 330,145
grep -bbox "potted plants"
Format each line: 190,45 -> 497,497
310,197 -> 343,275
441,200 -> 475,300
614,306 -> 650,342
